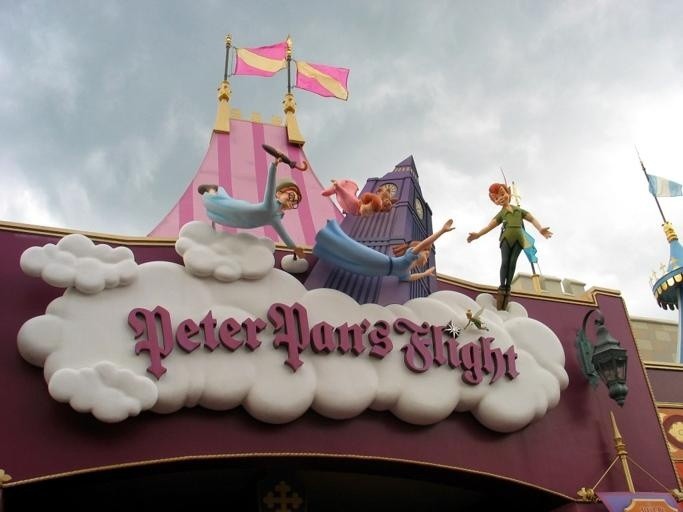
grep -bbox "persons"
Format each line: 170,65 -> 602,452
321,177 -> 393,217
466,183 -> 553,311
464,308 -> 490,332
198,156 -> 305,259
312,218 -> 456,282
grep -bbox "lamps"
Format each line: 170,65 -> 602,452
575,306 -> 631,412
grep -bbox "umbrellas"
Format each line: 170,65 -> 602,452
261,142 -> 308,171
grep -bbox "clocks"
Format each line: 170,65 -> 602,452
414,197 -> 424,221
377,183 -> 398,198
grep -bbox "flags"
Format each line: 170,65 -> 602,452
645,174 -> 683,197
291,57 -> 350,101
228,41 -> 288,78
